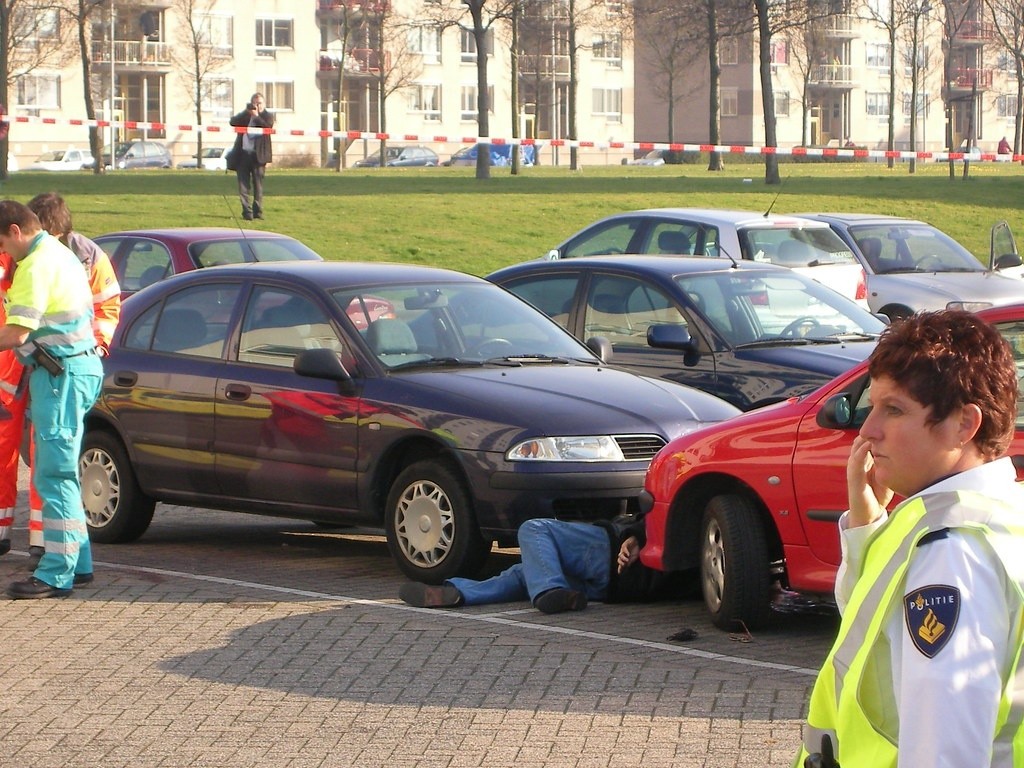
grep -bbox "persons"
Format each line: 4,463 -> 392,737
796,310 -> 1024,768
0,192 -> 121,599
399,510 -> 680,614
230,92 -> 273,222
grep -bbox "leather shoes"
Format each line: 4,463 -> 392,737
73,573 -> 94,587
6,576 -> 73,598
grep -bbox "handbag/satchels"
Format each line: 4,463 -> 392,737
225,152 -> 240,174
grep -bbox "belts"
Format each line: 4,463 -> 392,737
52,348 -> 94,359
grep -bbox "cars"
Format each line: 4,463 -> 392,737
80,141 -> 172,169
176,146 -> 232,171
18,147 -> 94,170
534,206 -> 870,335
630,149 -> 665,165
72,226 -> 395,376
342,254 -> 890,413
639,303 -> 1024,629
70,261 -> 752,584
355,145 -> 440,167
785,214 -> 1024,339
937,146 -> 985,162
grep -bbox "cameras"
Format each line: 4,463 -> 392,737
247,103 -> 257,110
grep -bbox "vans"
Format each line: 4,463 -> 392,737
441,144 -> 537,166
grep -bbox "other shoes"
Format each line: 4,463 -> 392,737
399,581 -> 464,608
257,217 -> 264,220
536,588 -> 587,613
0,539 -> 11,555
27,546 -> 45,570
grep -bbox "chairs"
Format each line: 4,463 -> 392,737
138,266 -> 170,290
776,239 -> 811,264
357,319 -> 434,367
859,237 -> 883,266
666,293 -> 725,339
591,280 -> 632,332
153,309 -> 208,349
657,231 -> 691,254
